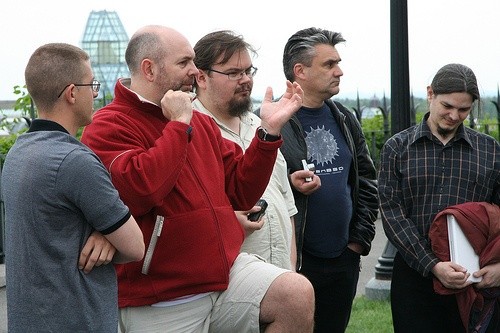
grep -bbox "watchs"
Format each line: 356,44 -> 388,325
258,126 -> 282,141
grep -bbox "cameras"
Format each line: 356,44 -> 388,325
301,159 -> 315,182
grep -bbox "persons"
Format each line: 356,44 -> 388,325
253,26 -> 380,332
378,63 -> 500,332
2,42 -> 145,333
187,29 -> 300,271
79,24 -> 316,332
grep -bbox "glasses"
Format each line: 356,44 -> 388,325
197,64 -> 258,82
58,80 -> 101,99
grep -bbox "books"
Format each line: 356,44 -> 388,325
448,213 -> 483,283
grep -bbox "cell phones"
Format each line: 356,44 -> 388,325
247,200 -> 268,222
